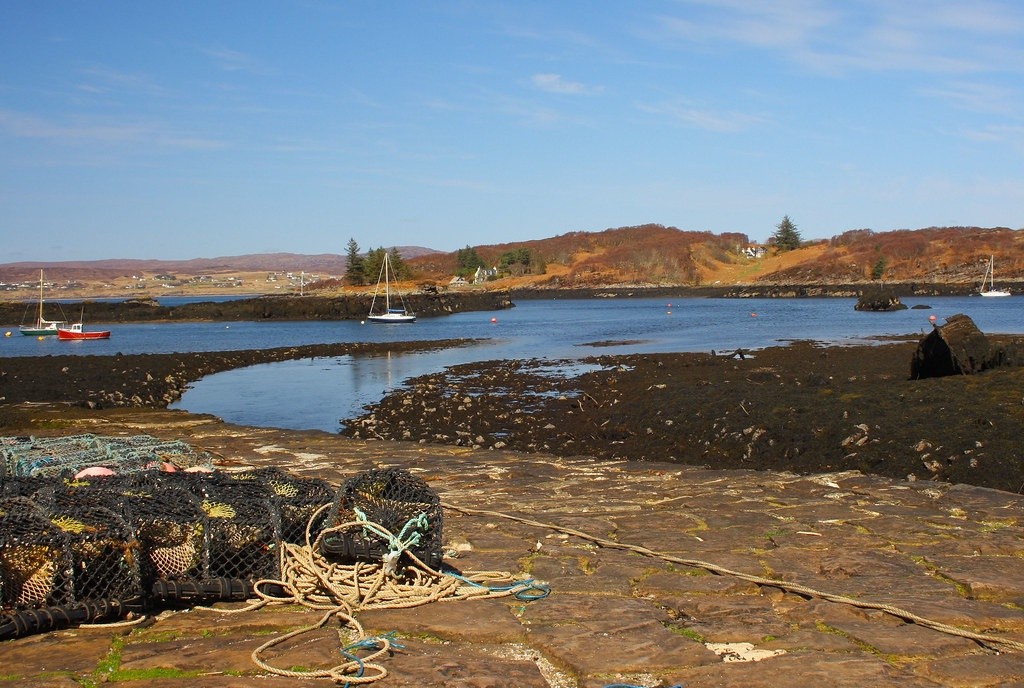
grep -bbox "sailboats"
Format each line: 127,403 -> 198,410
19,269 -> 69,335
979,255 -> 1011,297
366,253 -> 417,323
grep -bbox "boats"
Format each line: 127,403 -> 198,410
58,307 -> 111,341
